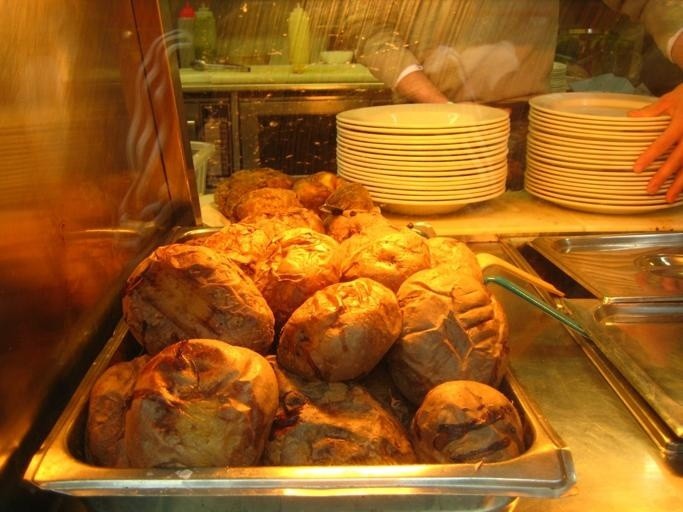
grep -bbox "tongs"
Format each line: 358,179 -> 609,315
189,53 -> 251,74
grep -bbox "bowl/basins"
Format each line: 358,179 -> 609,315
321,51 -> 355,67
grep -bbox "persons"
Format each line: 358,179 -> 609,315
343,1 -> 683,204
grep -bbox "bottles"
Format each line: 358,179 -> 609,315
176,1 -> 215,67
288,3 -> 310,66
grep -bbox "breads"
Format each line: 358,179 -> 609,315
88,167 -> 526,467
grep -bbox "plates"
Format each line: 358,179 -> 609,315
335,105 -> 511,215
523,91 -> 683,214
549,60 -> 567,90
